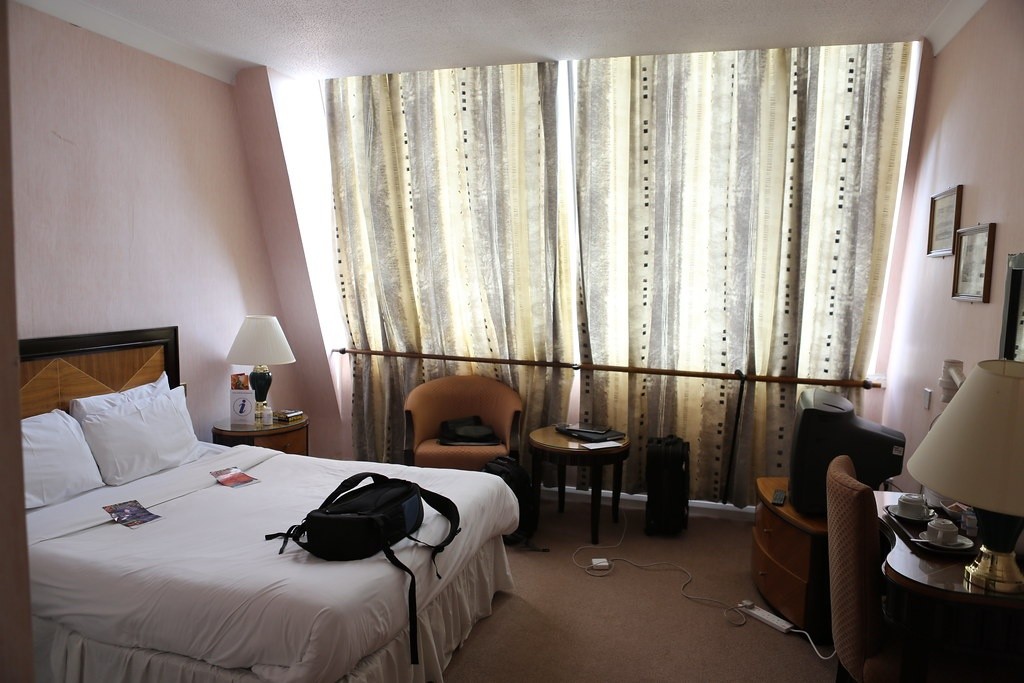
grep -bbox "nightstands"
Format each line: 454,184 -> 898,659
212,411 -> 309,454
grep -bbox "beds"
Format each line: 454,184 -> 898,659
18,326 -> 519,683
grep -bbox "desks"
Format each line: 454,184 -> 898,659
874,490 -> 1024,683
527,427 -> 632,545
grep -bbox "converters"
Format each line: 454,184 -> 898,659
592,559 -> 609,570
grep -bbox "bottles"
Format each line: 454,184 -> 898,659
263,407 -> 273,426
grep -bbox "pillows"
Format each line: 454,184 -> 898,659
69,369 -> 173,420
80,386 -> 203,484
22,409 -> 107,507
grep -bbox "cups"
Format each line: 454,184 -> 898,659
898,493 -> 929,519
926,518 -> 958,544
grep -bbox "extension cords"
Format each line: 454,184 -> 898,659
737,604 -> 795,633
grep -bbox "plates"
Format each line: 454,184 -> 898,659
919,531 -> 974,549
888,505 -> 938,521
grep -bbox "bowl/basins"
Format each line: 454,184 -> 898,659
940,500 -> 975,518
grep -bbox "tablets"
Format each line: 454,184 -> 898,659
565,422 -> 611,433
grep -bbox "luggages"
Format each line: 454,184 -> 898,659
645,435 -> 689,538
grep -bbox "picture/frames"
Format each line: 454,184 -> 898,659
926,184 -> 964,258
952,222 -> 996,304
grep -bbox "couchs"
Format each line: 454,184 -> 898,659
403,374 -> 527,480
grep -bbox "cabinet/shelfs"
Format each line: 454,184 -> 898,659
749,475 -> 839,632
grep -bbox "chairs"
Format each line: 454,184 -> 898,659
829,454 -> 967,683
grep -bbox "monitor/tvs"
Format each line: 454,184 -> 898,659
788,386 -> 906,521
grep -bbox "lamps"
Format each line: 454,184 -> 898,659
226,315 -> 296,418
905,357 -> 1024,593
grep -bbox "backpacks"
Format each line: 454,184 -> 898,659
265,470 -> 461,581
480,455 -> 541,546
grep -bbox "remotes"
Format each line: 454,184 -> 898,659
771,489 -> 786,506
